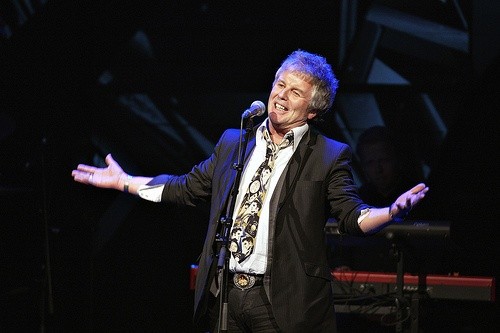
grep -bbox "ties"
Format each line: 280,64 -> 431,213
229,126 -> 295,261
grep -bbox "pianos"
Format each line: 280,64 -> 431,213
325,217 -> 452,240
191,264 -> 495,302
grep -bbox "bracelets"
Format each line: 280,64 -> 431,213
124,174 -> 133,193
388,202 -> 411,222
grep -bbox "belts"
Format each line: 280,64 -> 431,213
226,272 -> 264,289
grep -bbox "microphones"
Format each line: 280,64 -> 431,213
242,101 -> 266,118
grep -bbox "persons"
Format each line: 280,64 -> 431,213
323,125 -> 500,278
71,49 -> 429,333
